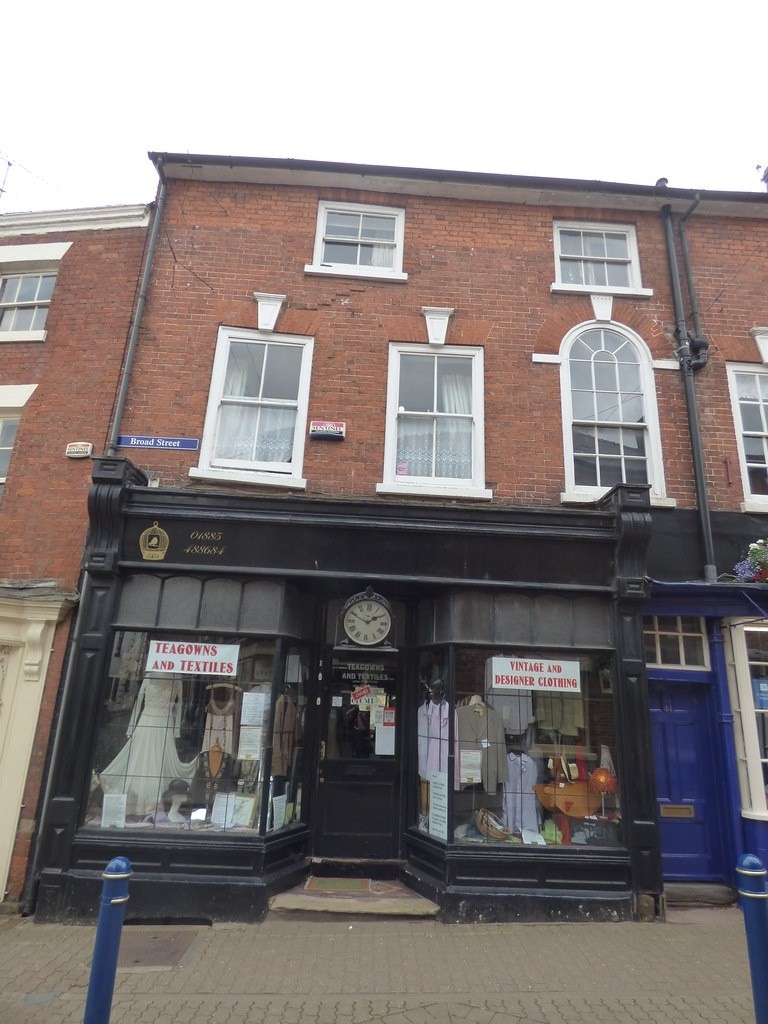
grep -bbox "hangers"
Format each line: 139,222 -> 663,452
425,688 -> 444,708
467,693 -> 485,712
206,681 -> 245,692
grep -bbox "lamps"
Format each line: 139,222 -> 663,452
589,767 -> 617,820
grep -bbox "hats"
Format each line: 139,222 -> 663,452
571,831 -> 587,845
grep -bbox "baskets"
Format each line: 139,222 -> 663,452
476,809 -> 512,840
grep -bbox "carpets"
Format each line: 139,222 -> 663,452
304,875 -> 371,893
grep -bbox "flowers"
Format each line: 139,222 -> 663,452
733,539 -> 768,583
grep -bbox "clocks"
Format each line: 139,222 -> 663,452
340,587 -> 395,646
